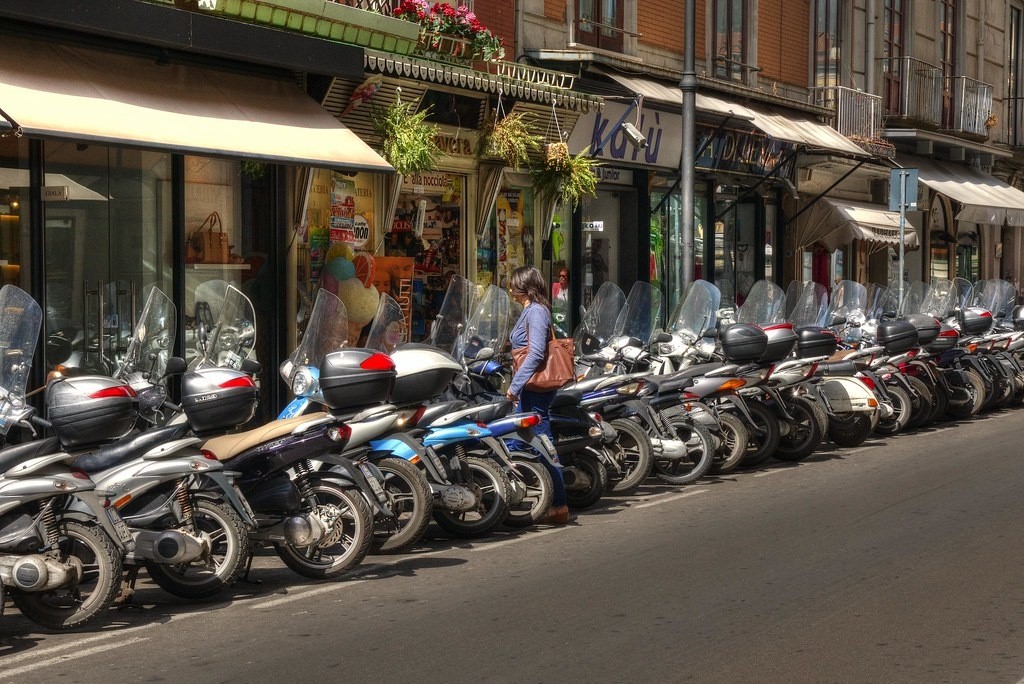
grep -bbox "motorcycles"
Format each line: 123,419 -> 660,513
75,284 -> 260,611
112,277 -> 1024,580
0,284 -> 141,631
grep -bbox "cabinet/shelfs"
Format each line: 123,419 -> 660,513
156,181 -> 255,343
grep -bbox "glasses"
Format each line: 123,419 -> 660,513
558,274 -> 566,278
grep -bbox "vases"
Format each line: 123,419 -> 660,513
416,30 -> 474,58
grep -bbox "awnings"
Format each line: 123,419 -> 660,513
1,46 -> 1024,247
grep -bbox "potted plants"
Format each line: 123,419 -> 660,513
475,87 -> 547,173
528,98 -> 609,212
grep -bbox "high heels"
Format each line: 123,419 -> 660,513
540,505 -> 570,527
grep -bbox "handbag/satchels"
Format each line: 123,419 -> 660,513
510,317 -> 574,389
185,211 -> 230,263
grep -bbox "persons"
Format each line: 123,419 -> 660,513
505,265 -> 569,524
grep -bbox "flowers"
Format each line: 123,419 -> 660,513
393,0 -> 505,61
